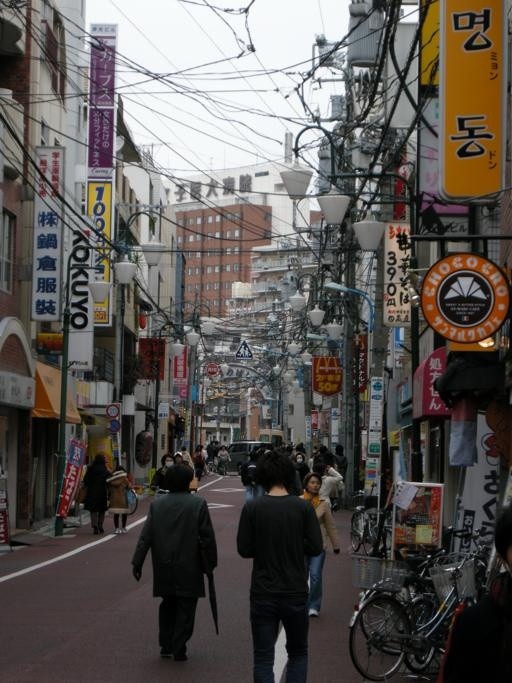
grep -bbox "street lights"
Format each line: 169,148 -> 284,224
280,123 -> 423,482
54,211 -> 165,538
288,272 -> 362,494
151,303 -> 216,494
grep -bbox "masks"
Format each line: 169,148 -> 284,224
297,459 -> 303,463
166,461 -> 174,468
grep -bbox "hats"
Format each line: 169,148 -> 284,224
173,451 -> 183,457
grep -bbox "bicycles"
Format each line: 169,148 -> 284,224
348,494 -> 494,682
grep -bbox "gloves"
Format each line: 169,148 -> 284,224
334,549 -> 340,554
133,565 -> 141,581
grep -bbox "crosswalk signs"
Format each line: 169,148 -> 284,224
235,341 -> 253,359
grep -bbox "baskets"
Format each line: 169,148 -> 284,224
350,554 -> 409,592
428,559 -> 476,601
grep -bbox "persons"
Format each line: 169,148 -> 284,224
297,473 -> 340,617
151,436 -> 231,494
437,506 -> 512,683
240,435 -> 348,512
236,452 -> 323,682
82,455 -> 132,535
131,462 -> 218,660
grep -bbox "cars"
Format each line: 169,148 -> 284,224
226,442 -> 275,475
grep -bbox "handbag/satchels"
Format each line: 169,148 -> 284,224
127,488 -> 134,504
78,484 -> 87,503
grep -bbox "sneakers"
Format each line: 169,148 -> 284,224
93,527 -> 128,534
308,608 -> 319,617
160,647 -> 173,656
332,500 -> 338,510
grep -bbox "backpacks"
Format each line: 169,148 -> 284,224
247,462 -> 257,481
